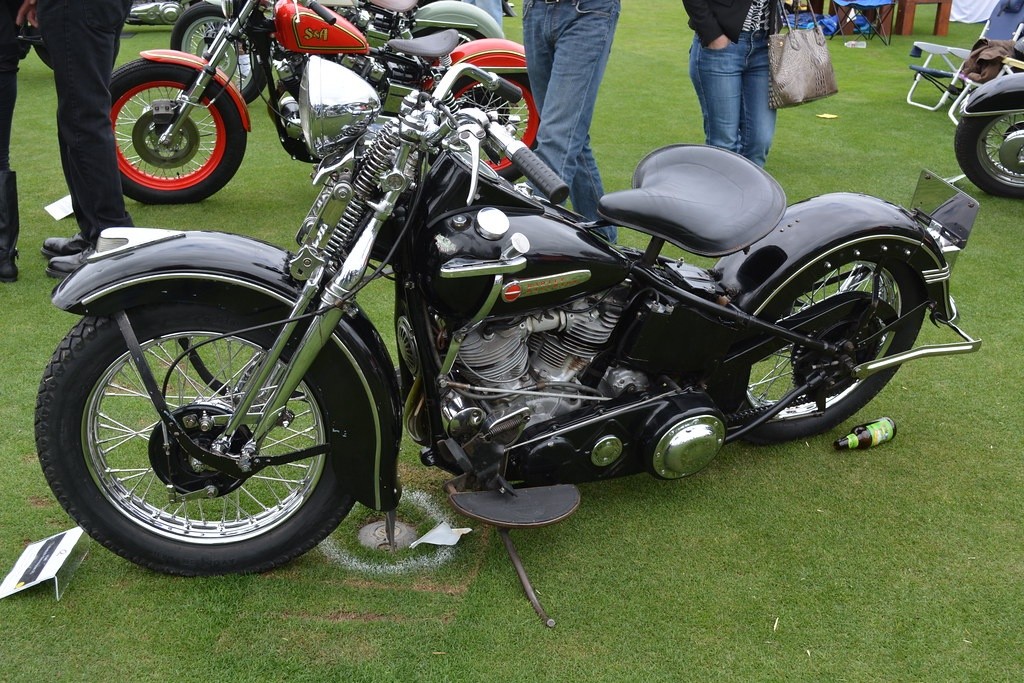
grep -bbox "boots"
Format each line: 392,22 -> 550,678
1,172 -> 19,283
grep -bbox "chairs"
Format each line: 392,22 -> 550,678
906,0 -> 1024,111
829,0 -> 896,49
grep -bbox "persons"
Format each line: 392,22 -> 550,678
459,0 -> 503,33
521,0 -> 620,247
0,0 -> 28,282
681,0 -> 785,171
16,0 -> 137,279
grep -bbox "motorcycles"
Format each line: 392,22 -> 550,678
954,72 -> 1024,201
33,61 -> 983,579
170,0 -> 505,106
108,0 -> 541,206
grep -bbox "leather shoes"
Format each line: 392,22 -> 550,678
46,245 -> 99,278
41,232 -> 92,259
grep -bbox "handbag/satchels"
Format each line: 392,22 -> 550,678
764,0 -> 839,108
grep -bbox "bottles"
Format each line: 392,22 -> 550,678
834,414 -> 897,451
